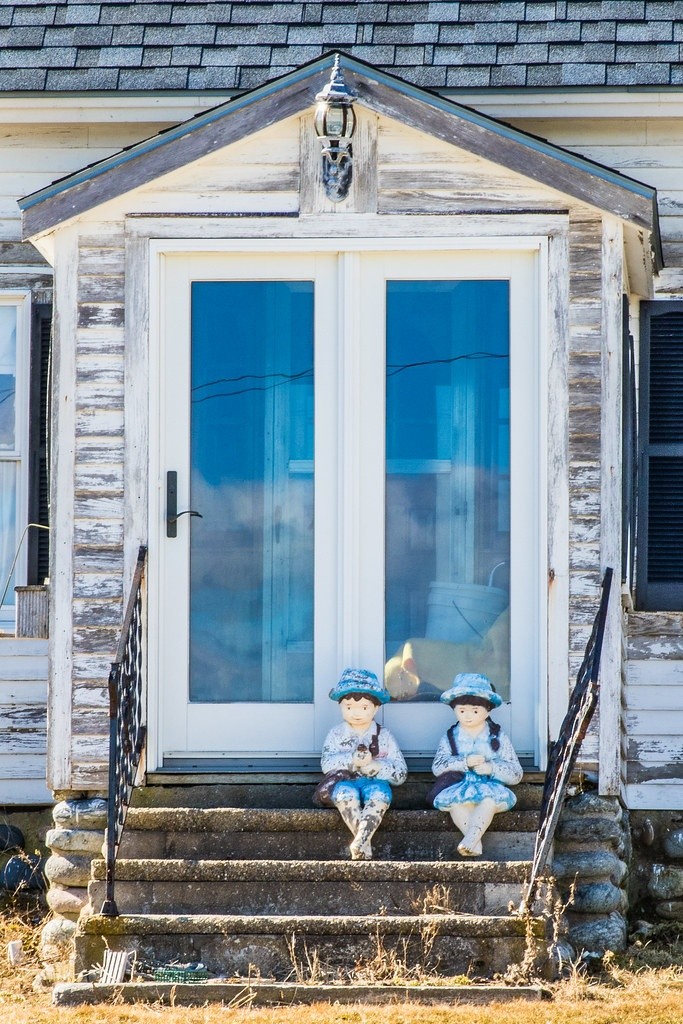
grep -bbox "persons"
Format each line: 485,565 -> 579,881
320,668 -> 407,860
432,673 -> 523,856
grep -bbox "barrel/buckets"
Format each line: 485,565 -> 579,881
427,580 -> 508,648
